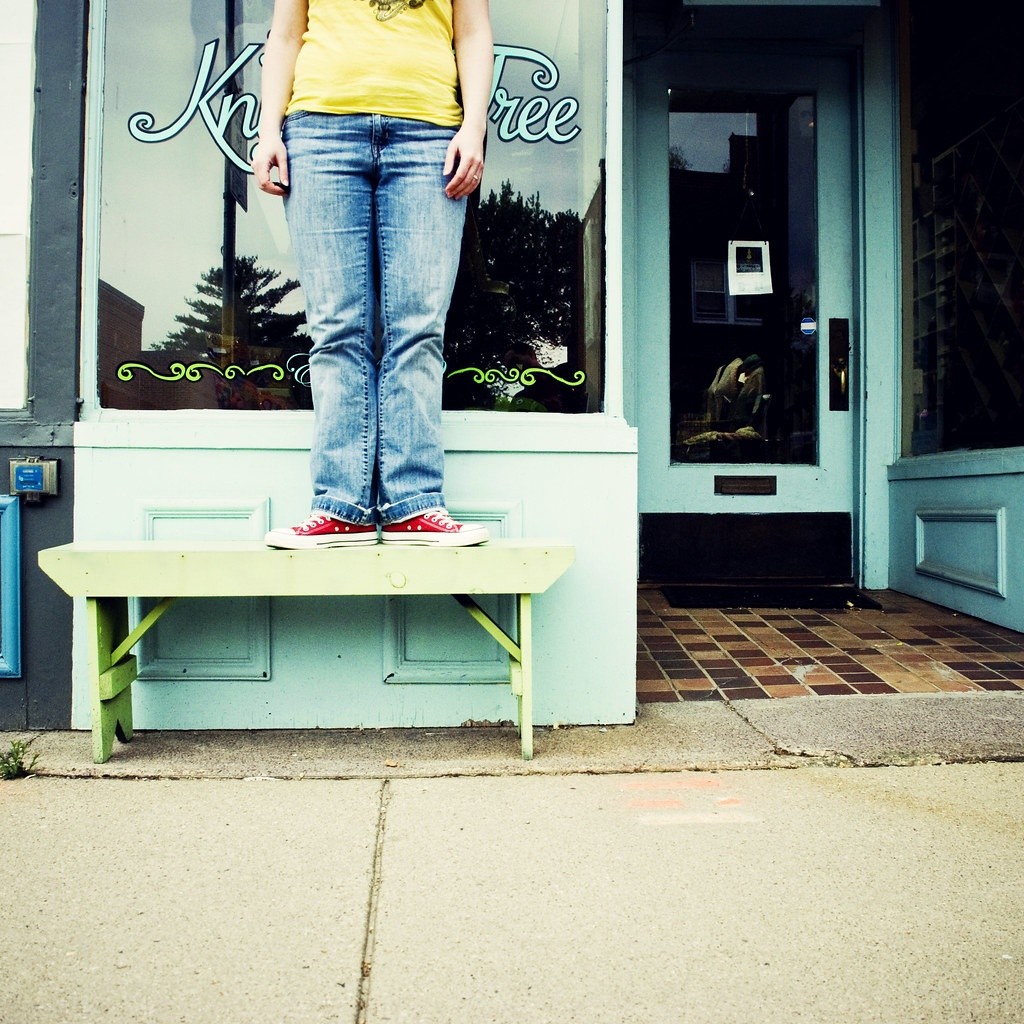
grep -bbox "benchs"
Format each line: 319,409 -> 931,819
35,531 -> 585,770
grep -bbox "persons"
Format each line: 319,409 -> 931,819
252,0 -> 495,548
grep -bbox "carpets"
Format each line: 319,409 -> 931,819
658,578 -> 883,612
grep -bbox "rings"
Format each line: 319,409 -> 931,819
474,175 -> 478,181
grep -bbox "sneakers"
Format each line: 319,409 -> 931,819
265,514 -> 379,549
381,510 -> 489,548
